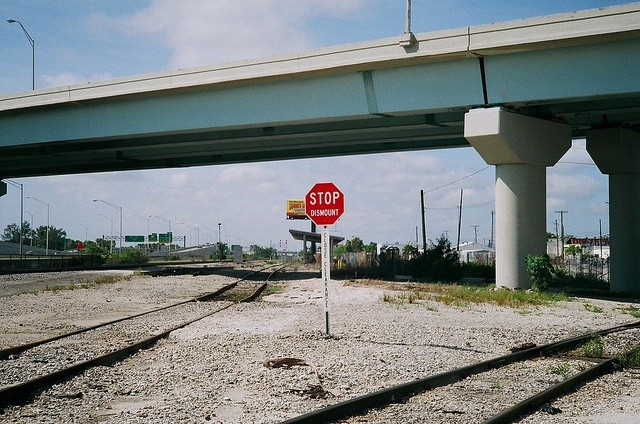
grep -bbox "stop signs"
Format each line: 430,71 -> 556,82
77,242 -> 84,249
304,182 -> 345,227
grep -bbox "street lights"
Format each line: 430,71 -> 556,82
149,213 -> 171,255
93,198 -> 122,252
27,195 -> 49,254
7,17 -> 36,91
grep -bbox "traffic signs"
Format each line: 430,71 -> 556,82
159,233 -> 169,242
167,231 -> 173,243
125,235 -> 145,243
148,235 -> 157,242
152,233 -> 157,236
